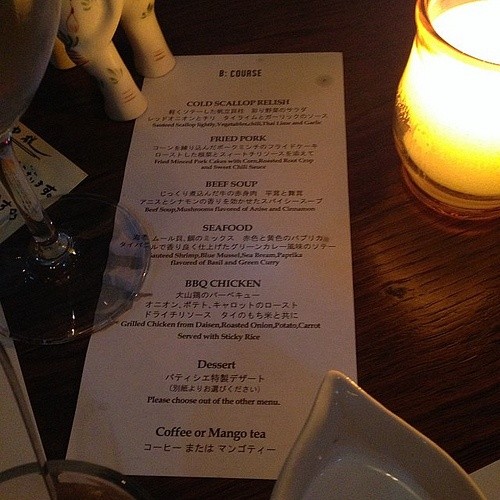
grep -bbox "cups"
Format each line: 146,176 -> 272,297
0,457 -> 157,500
390,0 -> 500,222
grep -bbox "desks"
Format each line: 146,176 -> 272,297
0,0 -> 499,500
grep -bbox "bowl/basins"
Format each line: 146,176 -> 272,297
270,369 -> 488,500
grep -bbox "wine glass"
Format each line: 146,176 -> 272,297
0,0 -> 152,345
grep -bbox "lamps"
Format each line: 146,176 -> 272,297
392,0 -> 500,222
